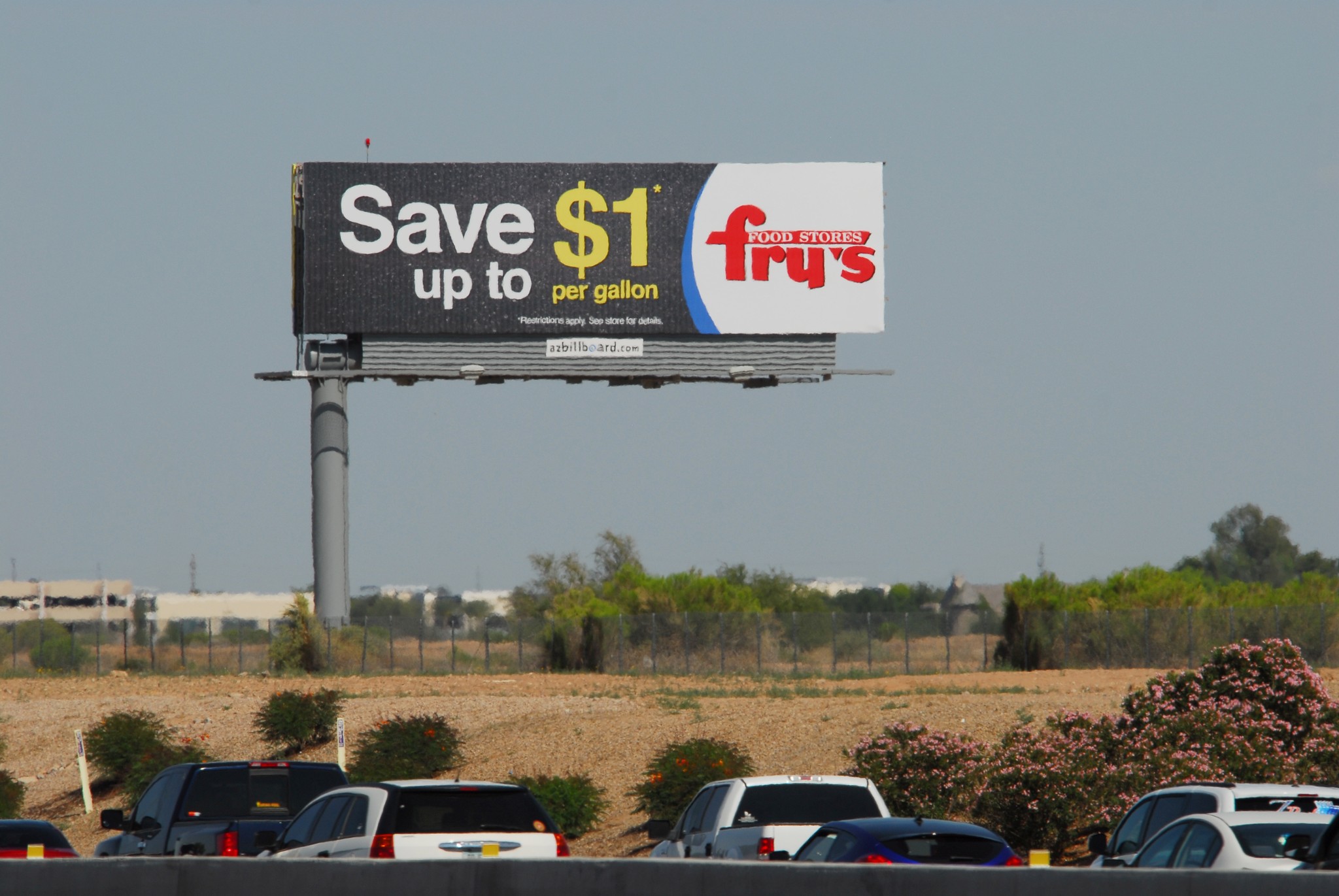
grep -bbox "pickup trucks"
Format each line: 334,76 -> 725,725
93,760 -> 351,858
649,774 -> 895,861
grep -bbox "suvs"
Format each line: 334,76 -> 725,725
1086,780 -> 1339,869
253,769 -> 571,860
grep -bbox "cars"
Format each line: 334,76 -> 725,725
767,813 -> 1024,867
1281,811 -> 1339,871
1102,812 -> 1336,872
0,819 -> 81,860
